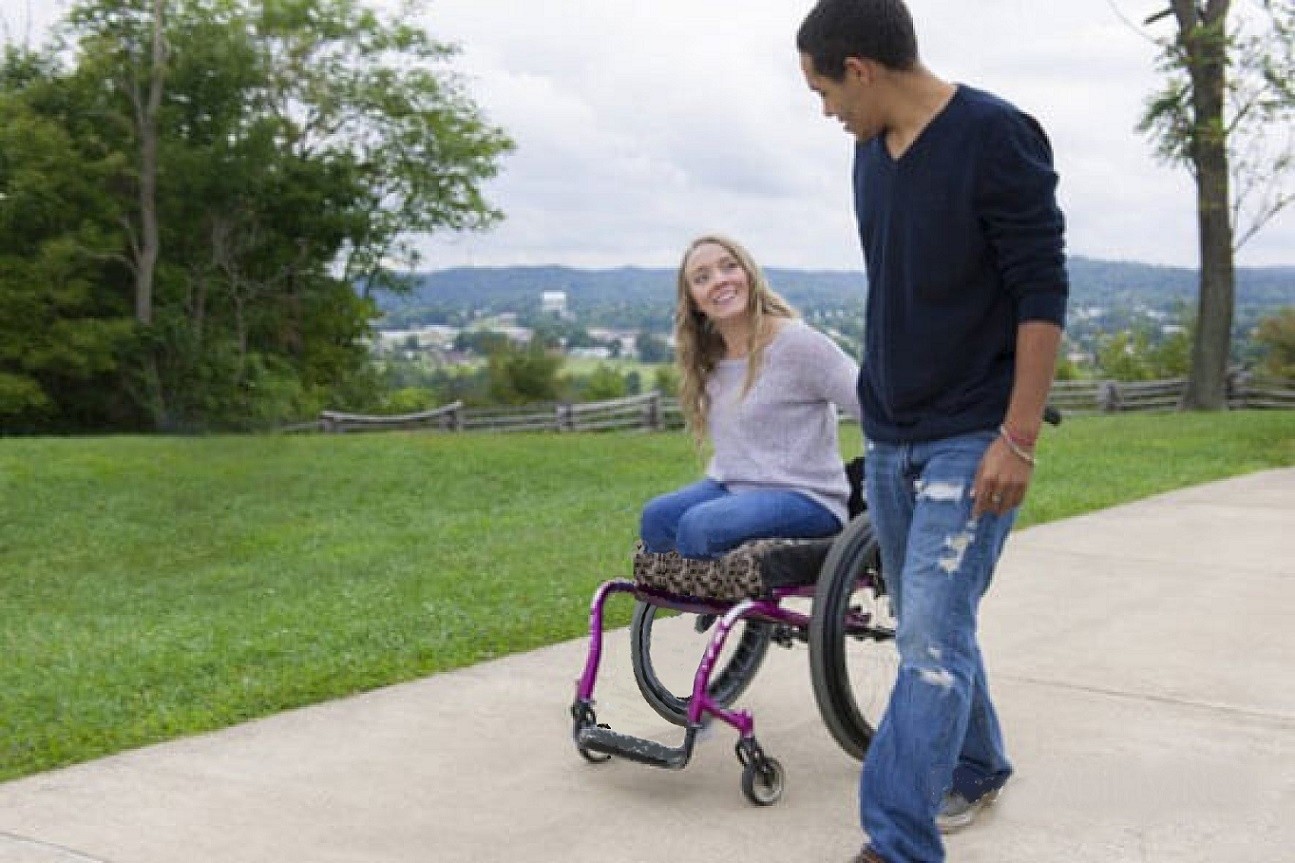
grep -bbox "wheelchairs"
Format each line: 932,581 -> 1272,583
568,406 -> 1062,808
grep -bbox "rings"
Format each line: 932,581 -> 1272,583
991,493 -> 1004,504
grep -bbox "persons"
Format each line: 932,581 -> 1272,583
797,0 -> 1070,863
641,236 -> 862,560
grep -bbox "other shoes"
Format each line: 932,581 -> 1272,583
934,784 -> 996,833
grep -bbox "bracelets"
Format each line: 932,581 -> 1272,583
1006,426 -> 1037,446
997,425 -> 1038,466
